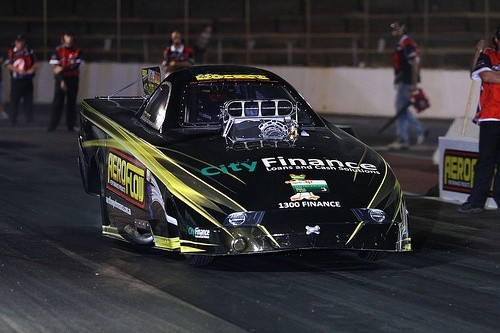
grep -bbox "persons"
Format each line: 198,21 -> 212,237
3,34 -> 38,124
385,19 -> 425,150
459,32 -> 500,212
46,32 -> 83,134
161,30 -> 194,79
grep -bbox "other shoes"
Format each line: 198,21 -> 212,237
387,143 -> 408,150
417,130 -> 428,145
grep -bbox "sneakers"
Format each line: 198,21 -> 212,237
458,202 -> 483,213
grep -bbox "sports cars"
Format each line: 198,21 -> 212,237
77,64 -> 413,268
